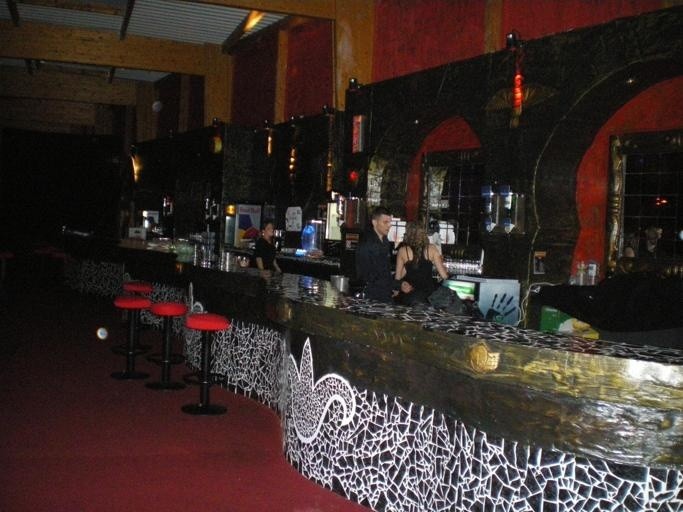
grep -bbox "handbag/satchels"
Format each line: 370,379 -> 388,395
427,284 -> 467,316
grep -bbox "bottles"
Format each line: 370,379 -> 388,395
574,259 -> 601,289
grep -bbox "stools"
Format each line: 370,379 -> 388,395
110,277 -> 234,420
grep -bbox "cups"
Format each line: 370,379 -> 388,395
236,255 -> 250,269
441,257 -> 481,271
328,273 -> 348,296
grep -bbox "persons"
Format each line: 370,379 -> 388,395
146,216 -> 158,232
393,220 -> 449,316
352,205 -> 415,306
622,223 -> 662,259
426,220 -> 443,256
251,220 -> 284,274
